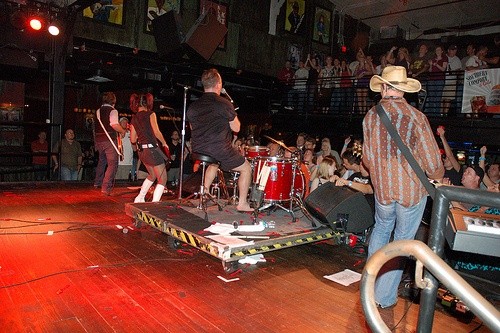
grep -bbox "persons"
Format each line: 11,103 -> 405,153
288,1 -> 325,43
436,127 -> 500,215
166,129 -> 181,190
90,0 -> 167,32
82,143 -> 99,179
187,69 -> 254,211
277,43 -> 500,116
93,92 -> 126,193
232,132 -> 375,214
362,66 -> 445,311
31,130 -> 48,181
115,117 -> 137,179
52,127 -> 82,181
130,92 -> 169,203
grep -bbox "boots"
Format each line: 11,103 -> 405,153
134,179 -> 153,203
152,184 -> 165,202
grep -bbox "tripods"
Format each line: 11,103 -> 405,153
228,173 -> 240,204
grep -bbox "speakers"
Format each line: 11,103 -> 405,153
177,171 -> 203,196
304,181 -> 375,233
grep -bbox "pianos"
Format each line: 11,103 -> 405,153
435,187 -> 500,262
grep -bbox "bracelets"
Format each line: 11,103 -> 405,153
77,164 -> 81,165
479,157 -> 485,160
349,181 -> 352,186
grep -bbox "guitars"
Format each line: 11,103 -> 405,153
164,146 -> 172,159
116,132 -> 125,161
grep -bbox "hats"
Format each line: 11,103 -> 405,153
120,117 -> 128,122
370,65 -> 421,93
465,160 -> 484,179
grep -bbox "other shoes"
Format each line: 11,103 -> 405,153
94,184 -> 100,189
102,190 -> 120,195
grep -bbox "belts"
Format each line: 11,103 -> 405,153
142,144 -> 159,148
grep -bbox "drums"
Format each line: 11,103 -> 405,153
245,146 -> 270,162
294,163 -> 310,199
255,157 -> 305,203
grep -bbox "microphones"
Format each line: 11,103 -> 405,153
221,88 -> 234,103
159,105 -> 174,110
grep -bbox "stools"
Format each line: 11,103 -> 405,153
176,152 -> 222,223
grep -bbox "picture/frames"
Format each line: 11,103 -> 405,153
311,5 -> 333,47
80,0 -> 127,28
143,0 -> 184,35
196,0 -> 229,51
283,0 -> 308,37
